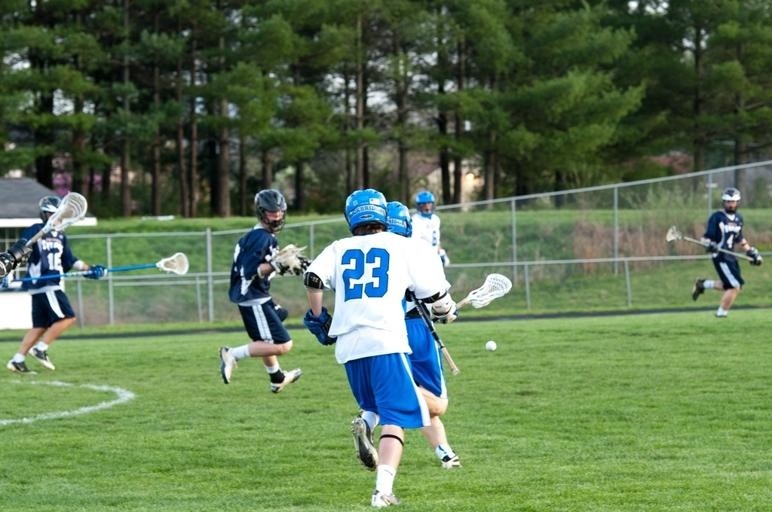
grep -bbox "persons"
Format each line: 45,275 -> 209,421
7,196 -> 108,375
303,189 -> 460,508
387,201 -> 462,468
691,188 -> 763,318
411,191 -> 450,268
219,189 -> 313,393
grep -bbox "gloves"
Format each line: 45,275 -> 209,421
746,247 -> 763,266
0,276 -> 9,288
304,307 -> 335,345
271,251 -> 310,276
705,241 -> 719,254
82,264 -> 106,279
431,301 -> 458,324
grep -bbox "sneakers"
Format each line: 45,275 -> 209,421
6,358 -> 38,375
371,489 -> 400,508
441,453 -> 461,471
692,278 -> 706,300
27,345 -> 57,369
350,416 -> 378,472
271,368 -> 302,394
220,345 -> 235,385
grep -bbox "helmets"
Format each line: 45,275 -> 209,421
344,189 -> 435,237
40,196 -> 60,220
254,189 -> 288,231
721,187 -> 741,213
